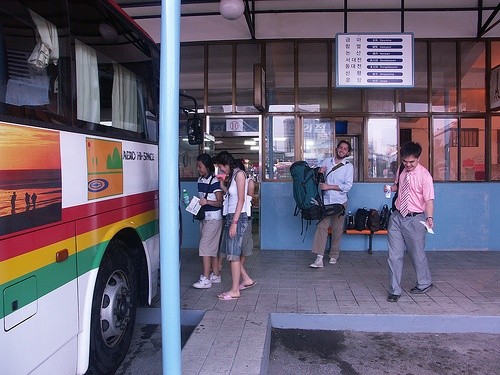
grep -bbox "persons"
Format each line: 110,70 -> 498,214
182,151 -> 256,301
310,140 -> 354,267
384,142 -> 435,301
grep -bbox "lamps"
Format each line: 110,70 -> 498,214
219,0 -> 244,20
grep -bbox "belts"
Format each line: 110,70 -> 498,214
396,209 -> 423,216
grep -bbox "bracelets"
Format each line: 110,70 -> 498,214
232,220 -> 238,224
426,217 -> 433,220
207,200 -> 208,204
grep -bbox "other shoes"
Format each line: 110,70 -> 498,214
310,255 -> 324,267
387,294 -> 401,302
328,258 -> 337,264
210,263 -> 223,272
410,284 -> 433,294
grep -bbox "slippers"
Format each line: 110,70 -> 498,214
217,292 -> 239,300
239,281 -> 257,290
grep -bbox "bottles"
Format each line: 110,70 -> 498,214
347,210 -> 355,227
182,189 -> 190,209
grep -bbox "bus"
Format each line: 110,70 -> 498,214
0,0 -> 206,375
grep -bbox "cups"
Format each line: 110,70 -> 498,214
385,185 -> 391,199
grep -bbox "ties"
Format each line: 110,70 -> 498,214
400,171 -> 410,217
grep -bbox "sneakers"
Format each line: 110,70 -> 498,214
209,272 -> 222,283
192,275 -> 212,288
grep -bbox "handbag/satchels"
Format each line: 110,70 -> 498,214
368,209 -> 380,232
392,165 -> 404,212
192,206 -> 205,220
355,207 -> 369,231
223,195 -> 228,216
380,204 -> 391,230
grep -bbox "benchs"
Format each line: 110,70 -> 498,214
326,227 -> 388,255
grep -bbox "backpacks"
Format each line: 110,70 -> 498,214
290,161 -> 326,220
